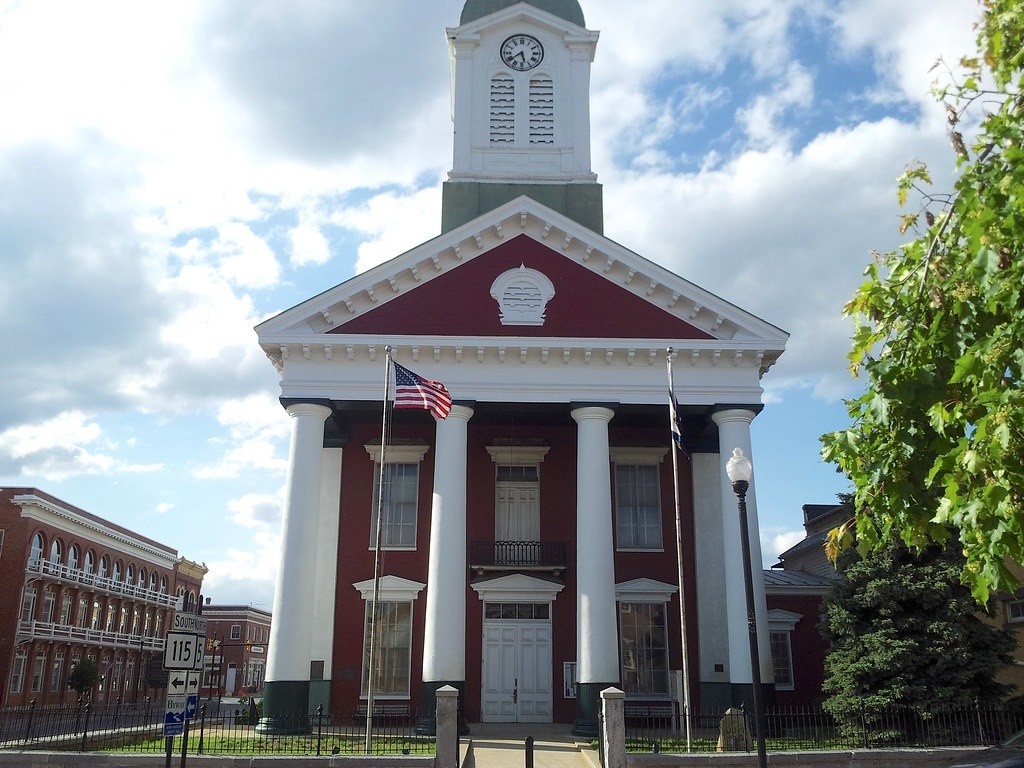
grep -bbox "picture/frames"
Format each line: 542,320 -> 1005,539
564,662 -> 578,698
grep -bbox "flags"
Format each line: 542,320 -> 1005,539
667,358 -> 693,460
391,362 -> 451,419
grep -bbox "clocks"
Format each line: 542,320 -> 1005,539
500,34 -> 544,71
